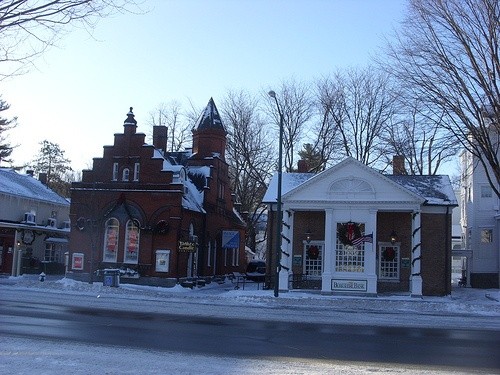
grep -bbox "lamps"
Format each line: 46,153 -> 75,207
304,210 -> 313,244
389,212 -> 398,245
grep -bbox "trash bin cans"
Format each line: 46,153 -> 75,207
101,268 -> 121,288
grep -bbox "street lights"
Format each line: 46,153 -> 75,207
268,90 -> 282,297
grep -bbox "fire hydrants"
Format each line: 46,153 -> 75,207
40,271 -> 45,283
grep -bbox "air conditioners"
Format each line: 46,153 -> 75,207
63,220 -> 71,230
47,218 -> 57,229
24,213 -> 36,225
